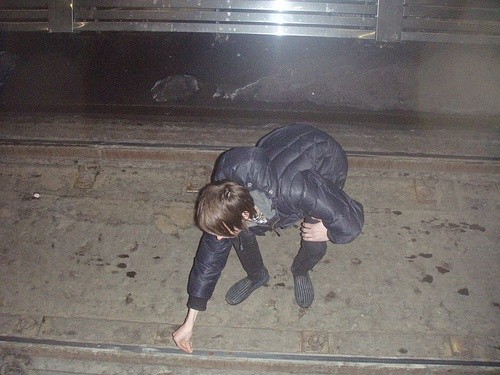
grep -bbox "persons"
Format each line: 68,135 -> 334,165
171,125 -> 365,354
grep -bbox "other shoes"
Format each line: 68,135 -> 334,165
291,263 -> 313,308
226,269 -> 268,304
